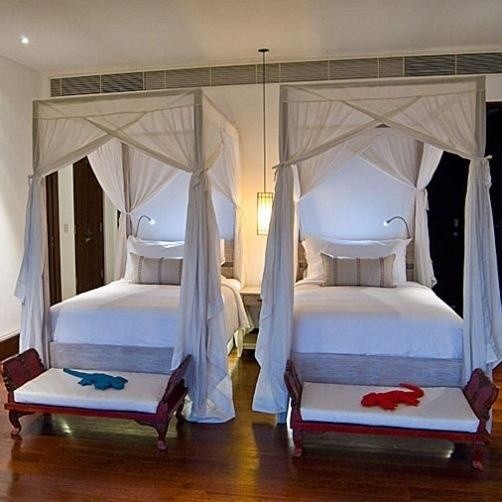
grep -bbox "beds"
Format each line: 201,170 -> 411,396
286,229 -> 464,386
44,240 -> 241,376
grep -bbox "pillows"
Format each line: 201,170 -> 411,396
301,237 -> 413,289
125,235 -> 185,284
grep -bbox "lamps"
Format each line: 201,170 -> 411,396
135,214 -> 153,237
384,215 -> 410,240
257,47 -> 274,238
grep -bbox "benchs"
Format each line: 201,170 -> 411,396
0,348 -> 193,451
283,358 -> 499,471
